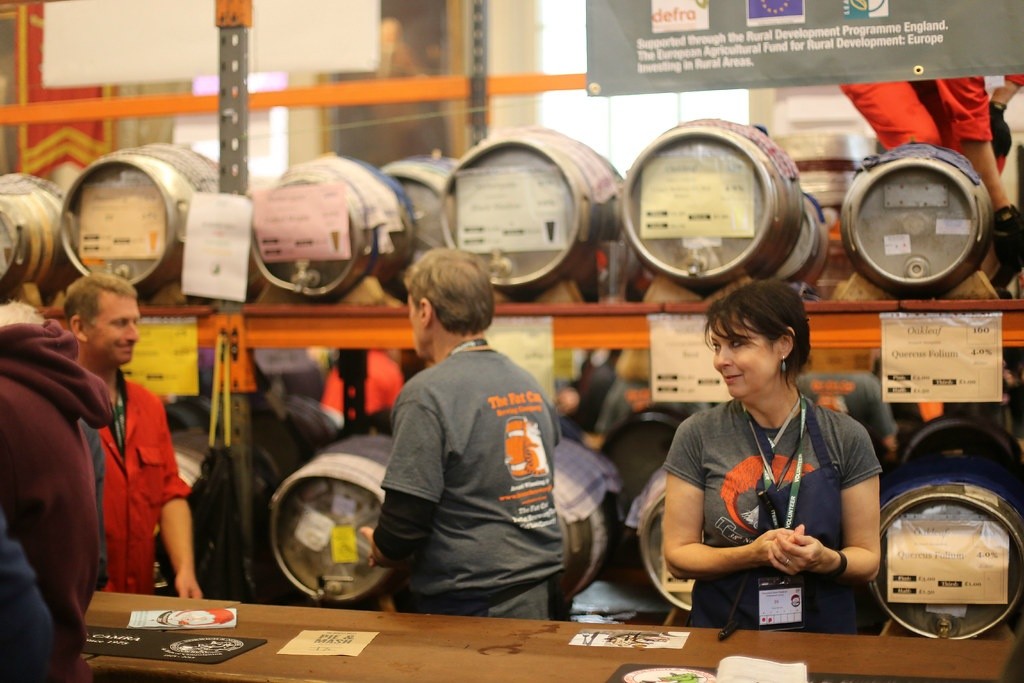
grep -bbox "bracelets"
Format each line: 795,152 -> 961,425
828,549 -> 847,576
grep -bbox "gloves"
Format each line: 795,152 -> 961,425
988,101 -> 1011,156
990,203 -> 1024,270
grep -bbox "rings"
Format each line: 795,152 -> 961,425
784,559 -> 789,566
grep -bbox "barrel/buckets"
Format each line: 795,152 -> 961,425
553,437 -> 622,604
621,120 -> 805,295
775,192 -> 828,291
840,142 -> 993,301
601,406 -> 694,542
268,432 -> 414,603
155,427 -> 283,587
164,392 -> 254,434
442,128 -> 627,300
640,469 -> 704,610
255,395 -> 339,479
865,470 -> 1024,640
61,142 -> 220,303
0,172 -> 78,304
896,412 -> 1023,481
377,155 -> 455,300
251,153 -> 417,300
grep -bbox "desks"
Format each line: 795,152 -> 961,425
82,590 -> 1018,683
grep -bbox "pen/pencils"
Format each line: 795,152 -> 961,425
718,619 -> 739,640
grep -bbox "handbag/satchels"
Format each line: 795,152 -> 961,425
157,444 -> 251,604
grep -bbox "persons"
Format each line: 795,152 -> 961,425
662,281 -> 883,633
64,274 -> 206,600
554,345 -> 1024,460
320,349 -> 404,429
359,247 -> 566,621
0,285 -> 115,683
838,74 -> 1024,274
